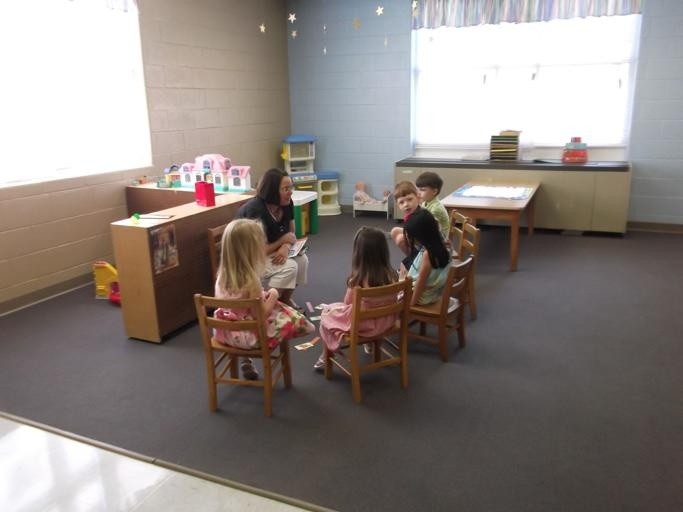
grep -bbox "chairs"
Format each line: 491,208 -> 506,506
447,209 -> 473,259
324,276 -> 412,402
453,224 -> 481,320
207,223 -> 228,281
409,253 -> 477,361
194,293 -> 292,418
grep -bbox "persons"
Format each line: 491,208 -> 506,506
399,207 -> 450,306
399,180 -> 447,278
212,218 -> 316,380
235,167 -> 309,317
391,172 -> 450,255
313,226 -> 399,369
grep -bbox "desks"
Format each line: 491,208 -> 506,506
439,179 -> 541,271
291,190 -> 319,239
393,157 -> 631,236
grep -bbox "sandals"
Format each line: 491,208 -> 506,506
314,356 -> 338,369
362,343 -> 376,355
239,358 -> 258,379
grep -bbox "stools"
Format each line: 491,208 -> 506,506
353,192 -> 393,221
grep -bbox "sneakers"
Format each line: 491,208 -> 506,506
277,297 -> 307,314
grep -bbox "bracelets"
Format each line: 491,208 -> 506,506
281,245 -> 290,251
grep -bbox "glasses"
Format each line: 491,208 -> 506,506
280,186 -> 295,192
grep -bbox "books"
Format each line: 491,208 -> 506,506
490,135 -> 519,160
